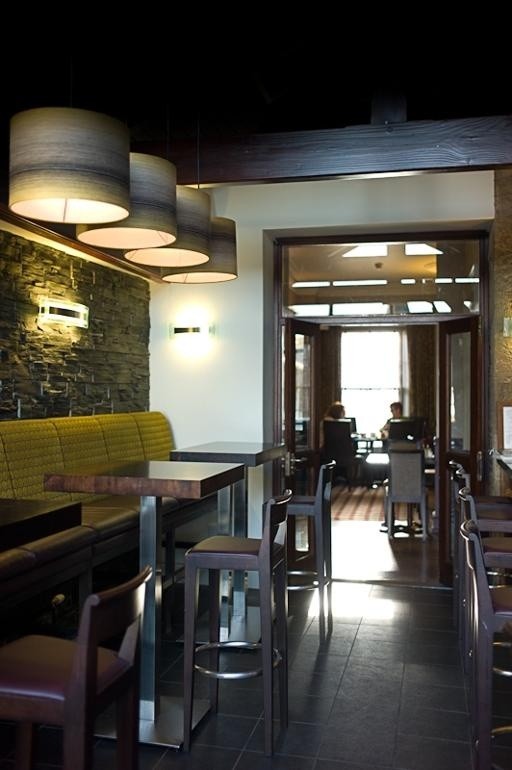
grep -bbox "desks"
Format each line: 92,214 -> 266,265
169,440 -> 287,648
43,459 -> 245,750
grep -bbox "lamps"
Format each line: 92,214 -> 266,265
8,107 -> 130,224
124,184 -> 211,268
75,152 -> 178,249
160,216 -> 239,284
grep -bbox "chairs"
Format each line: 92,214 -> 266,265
49,416 -> 139,539
455,487 -> 512,675
322,419 -> 362,492
97,413 -> 179,515
0,419 -> 95,556
457,519 -> 512,769
454,466 -> 512,552
0,439 -> 37,577
386,449 -> 428,541
262,460 -> 336,601
131,411 -> 175,461
181,489 -> 293,759
0,564 -> 153,770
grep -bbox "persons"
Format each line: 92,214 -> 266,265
379,402 -> 404,439
325,402 -> 346,420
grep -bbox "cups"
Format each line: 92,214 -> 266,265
371,432 -> 376,440
361,433 -> 366,438
